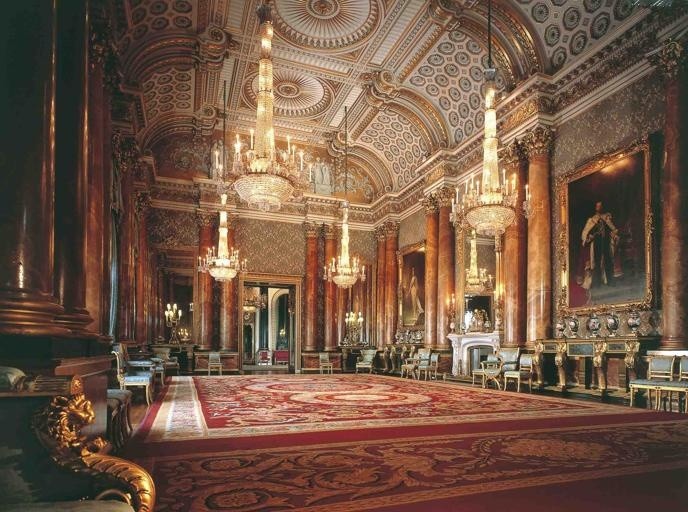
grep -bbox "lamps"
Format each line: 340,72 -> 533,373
195,0 -> 312,282
449,0 -> 532,239
464,230 -> 492,295
321,107 -> 366,290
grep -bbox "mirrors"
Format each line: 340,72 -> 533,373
461,224 -> 498,334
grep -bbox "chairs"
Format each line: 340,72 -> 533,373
111,342 -> 165,406
628,354 -> 688,415
208,351 -> 223,376
471,343 -> 535,394
400,348 -> 440,380
354,349 -> 377,374
319,351 -> 333,375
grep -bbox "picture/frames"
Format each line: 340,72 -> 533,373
395,239 -> 425,333
557,129 -> 656,317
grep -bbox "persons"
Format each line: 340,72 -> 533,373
580,197 -> 625,289
404,265 -> 424,322
